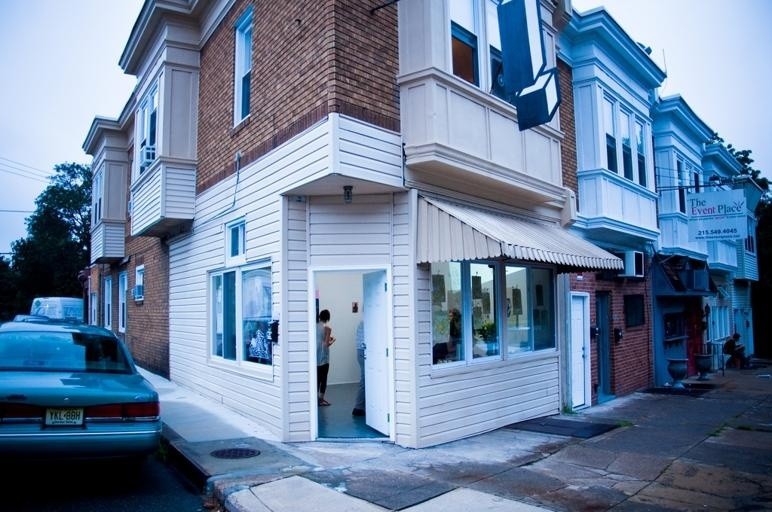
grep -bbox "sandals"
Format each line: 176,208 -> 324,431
319,400 -> 330,406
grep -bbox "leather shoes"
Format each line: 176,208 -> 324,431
352,408 -> 365,416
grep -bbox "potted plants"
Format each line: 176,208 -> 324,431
474,317 -> 498,356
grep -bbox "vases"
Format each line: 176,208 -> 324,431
694,355 -> 712,381
667,359 -> 689,389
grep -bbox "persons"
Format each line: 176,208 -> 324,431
723,333 -> 753,370
433,336 -> 454,363
473,328 -> 489,359
447,307 -> 462,352
317,310 -> 336,406
352,320 -> 366,416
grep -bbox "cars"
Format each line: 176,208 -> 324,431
1,310 -> 167,456
